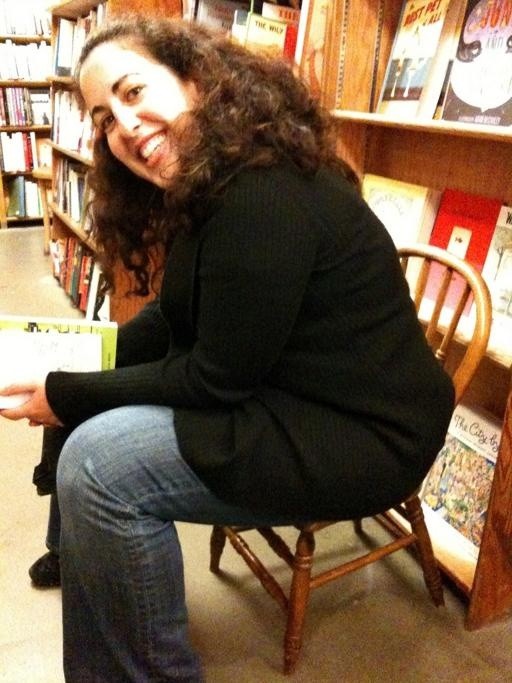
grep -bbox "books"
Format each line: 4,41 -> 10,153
222,0 -> 512,592
1,315 -> 120,410
3,0 -> 117,322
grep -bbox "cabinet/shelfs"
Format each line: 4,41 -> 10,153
45,1 -> 312,324
299,0 -> 510,629
0,35 -> 57,228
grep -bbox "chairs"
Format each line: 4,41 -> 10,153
209,245 -> 494,675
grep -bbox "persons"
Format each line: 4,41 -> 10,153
1,15 -> 457,681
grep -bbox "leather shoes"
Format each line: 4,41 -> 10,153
28,548 -> 61,588
